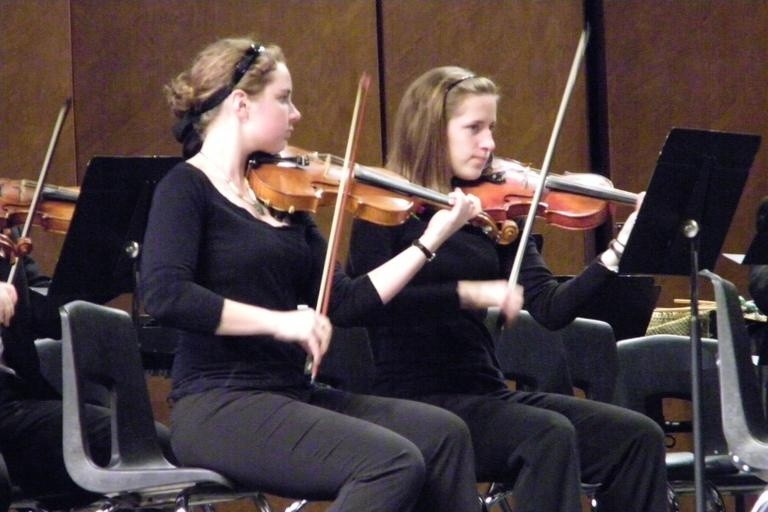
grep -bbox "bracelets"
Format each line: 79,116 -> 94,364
614,237 -> 627,248
607,239 -> 623,259
408,237 -> 437,264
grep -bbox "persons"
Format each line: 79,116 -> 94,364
137,32 -> 478,510
746,195 -> 768,317
1,205 -> 112,511
341,62 -> 672,511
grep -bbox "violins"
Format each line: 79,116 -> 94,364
2,176 -> 81,234
452,155 -> 639,230
246,144 -> 520,245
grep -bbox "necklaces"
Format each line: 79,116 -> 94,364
198,150 -> 265,215
